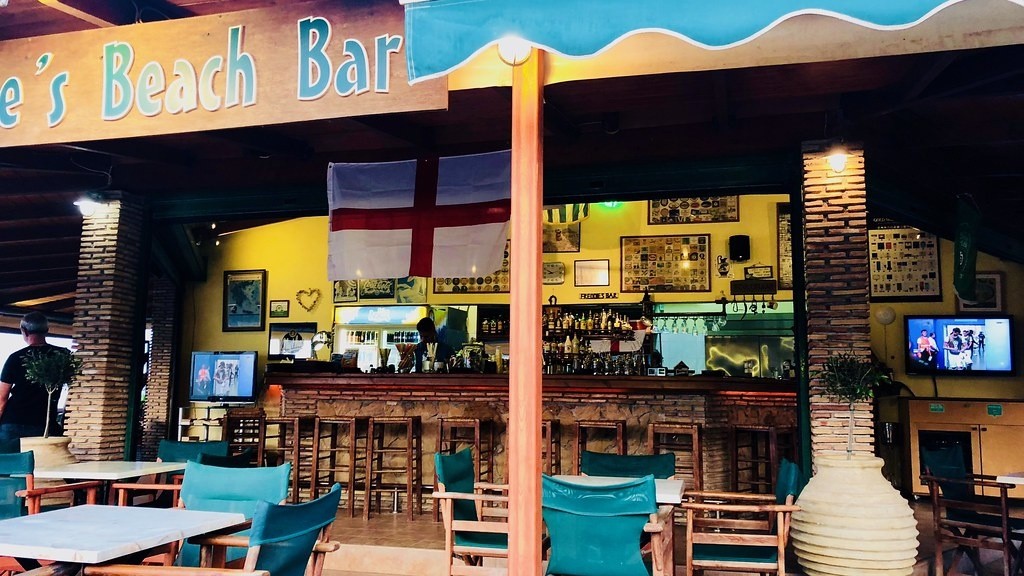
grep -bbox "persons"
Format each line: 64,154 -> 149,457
944,327 -> 978,370
978,332 -> 985,349
916,329 -> 939,370
414,317 -> 456,373
0,311 -> 73,452
197,361 -> 239,394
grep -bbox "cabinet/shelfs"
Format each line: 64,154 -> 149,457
896,396 -> 1024,503
178,404 -> 257,443
477,294 -> 654,365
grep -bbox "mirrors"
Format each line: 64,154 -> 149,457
574,259 -> 610,287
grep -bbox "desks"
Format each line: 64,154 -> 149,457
1,503 -> 246,576
11,461 -> 187,506
997,472 -> 1024,484
547,474 -> 686,576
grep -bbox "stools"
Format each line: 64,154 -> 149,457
730,422 -> 798,494
309,416 -> 368,518
573,419 -> 627,476
363,416 -> 422,522
501,418 -> 561,523
648,421 -> 705,503
433,416 -> 493,522
222,407 -> 318,505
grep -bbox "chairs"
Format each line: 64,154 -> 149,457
920,445 -> 1024,576
542,473 -> 665,576
0,440 -> 342,576
580,450 -> 675,481
433,448 -> 550,576
682,459 -> 804,576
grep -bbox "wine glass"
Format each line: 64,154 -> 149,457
379,348 -> 391,374
652,316 -> 726,336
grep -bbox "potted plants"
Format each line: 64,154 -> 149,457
19,345 -> 95,505
789,351 -> 921,576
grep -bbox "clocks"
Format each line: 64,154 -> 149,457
543,262 -> 565,285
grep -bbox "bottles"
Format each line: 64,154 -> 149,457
488,354 -> 509,374
422,353 -> 432,373
346,331 -> 379,346
592,352 -> 646,376
542,333 -> 592,375
483,315 -> 509,337
387,331 -> 420,343
342,350 -> 358,369
769,361 -> 795,380
745,361 -> 753,377
543,309 -> 632,335
370,365 -> 373,373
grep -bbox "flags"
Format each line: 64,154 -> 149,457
328,147 -> 511,280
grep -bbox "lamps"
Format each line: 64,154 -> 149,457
72,191 -> 104,216
498,39 -> 532,66
820,139 -> 855,174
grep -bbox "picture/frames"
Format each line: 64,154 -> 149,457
955,271 -> 1007,315
433,239 -> 510,293
620,233 -> 712,293
542,222 -> 581,253
269,300 -> 290,317
222,270 -> 266,332
745,266 -> 773,279
648,196 -> 740,225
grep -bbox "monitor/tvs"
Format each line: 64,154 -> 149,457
902,314 -> 1015,378
190,350 -> 259,403
